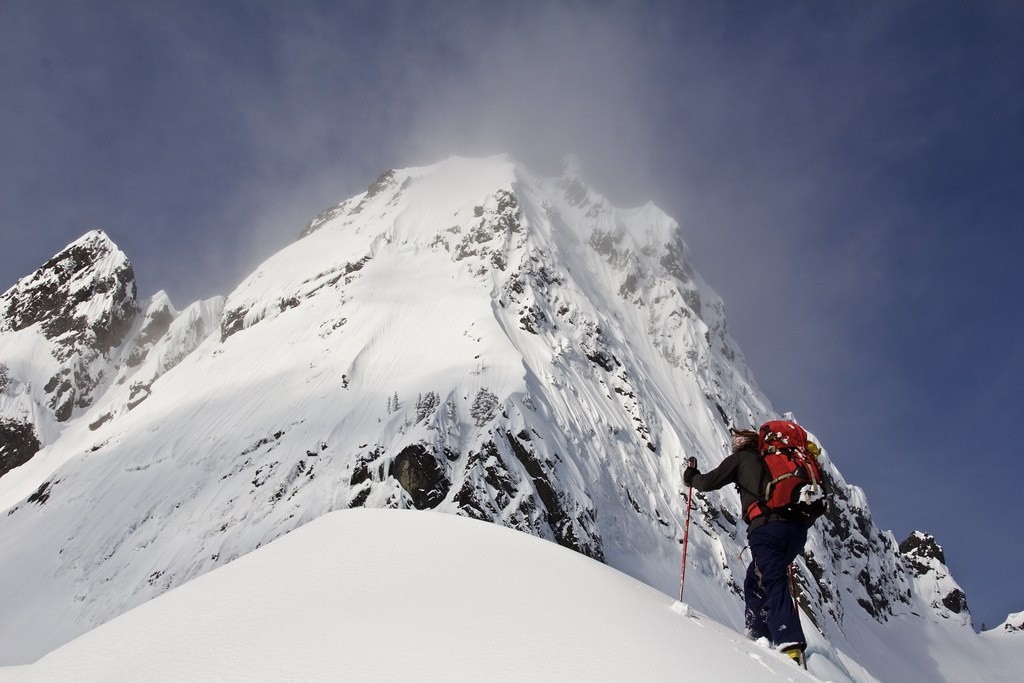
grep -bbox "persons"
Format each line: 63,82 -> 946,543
683,428 -> 808,665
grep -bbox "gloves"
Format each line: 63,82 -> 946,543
683,466 -> 700,487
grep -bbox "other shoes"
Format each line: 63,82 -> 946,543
779,641 -> 807,666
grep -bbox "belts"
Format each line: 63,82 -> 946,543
747,513 -> 786,534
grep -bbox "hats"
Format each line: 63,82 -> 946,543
729,426 -> 759,453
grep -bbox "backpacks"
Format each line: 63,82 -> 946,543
736,420 -> 829,526
